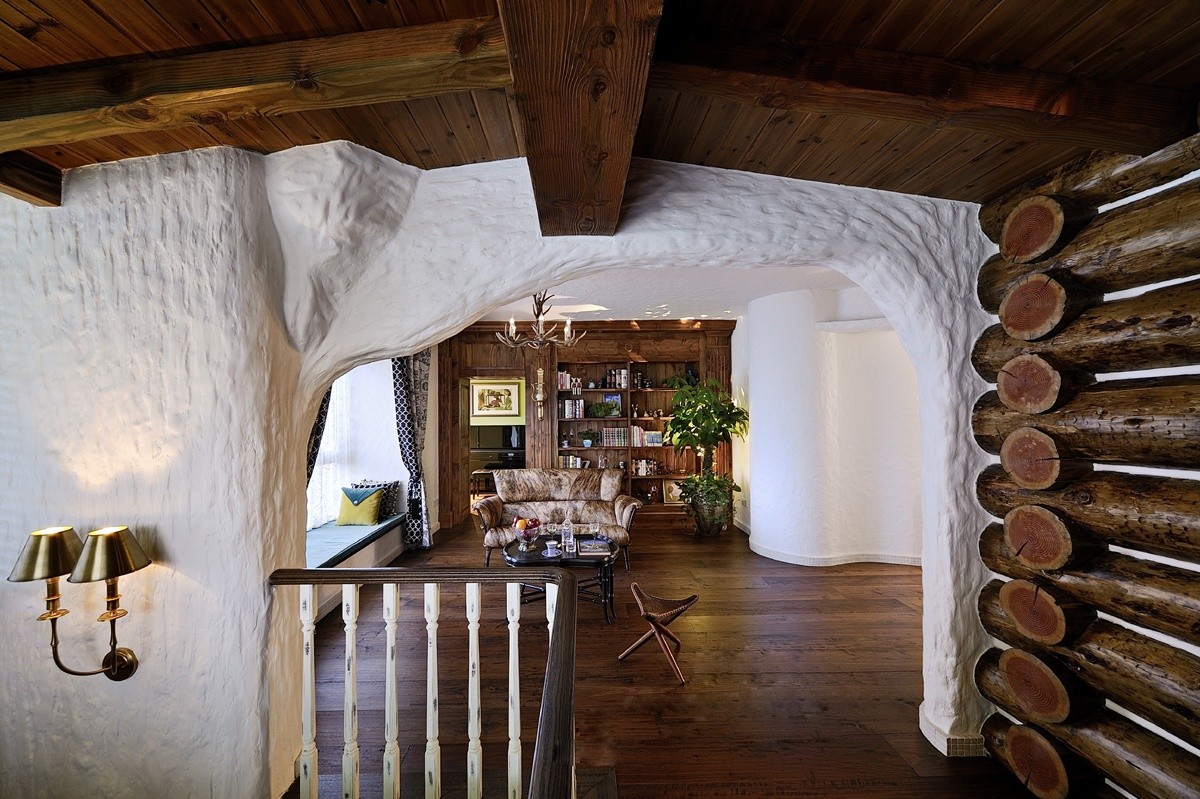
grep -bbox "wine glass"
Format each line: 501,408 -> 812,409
547,521 -> 556,540
590,519 -> 600,549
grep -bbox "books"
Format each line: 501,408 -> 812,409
631,458 -> 658,476
577,539 -> 611,556
557,371 -> 571,389
558,456 -> 582,468
602,427 -> 663,448
557,399 -> 585,418
605,369 -> 644,389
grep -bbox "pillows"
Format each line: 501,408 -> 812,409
359,480 -> 399,516
334,487 -> 384,526
351,483 -> 392,521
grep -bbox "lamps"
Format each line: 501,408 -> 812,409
530,368 -> 548,421
4,523 -> 151,681
493,289 -> 587,350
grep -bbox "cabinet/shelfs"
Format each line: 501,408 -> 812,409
555,359 -> 703,515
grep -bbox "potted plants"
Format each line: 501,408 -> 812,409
661,373 -> 752,541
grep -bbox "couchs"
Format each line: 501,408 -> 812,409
473,467 -> 642,570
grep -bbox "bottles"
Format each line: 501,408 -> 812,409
562,509 -> 573,545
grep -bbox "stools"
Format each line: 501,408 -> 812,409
617,581 -> 699,687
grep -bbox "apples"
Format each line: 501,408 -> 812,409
512,515 -> 540,540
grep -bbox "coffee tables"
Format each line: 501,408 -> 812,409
500,534 -> 620,625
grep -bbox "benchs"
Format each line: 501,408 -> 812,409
305,511 -> 407,623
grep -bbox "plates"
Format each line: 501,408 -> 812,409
542,549 -> 562,557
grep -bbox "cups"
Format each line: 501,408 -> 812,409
566,538 -> 576,553
545,540 -> 558,555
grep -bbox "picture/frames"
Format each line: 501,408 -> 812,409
471,382 -> 521,417
